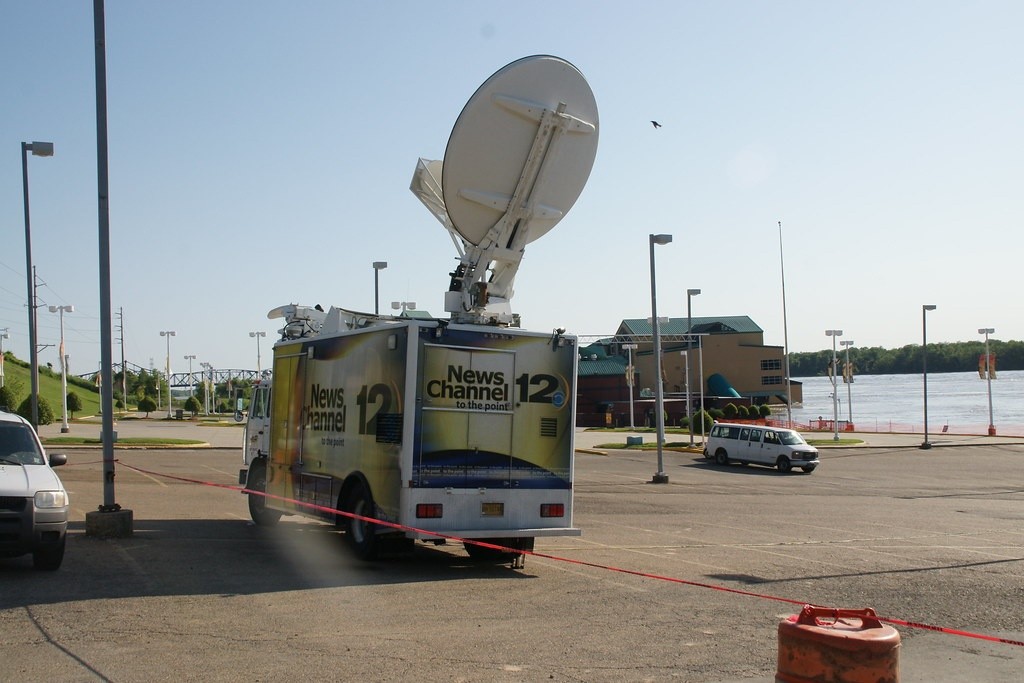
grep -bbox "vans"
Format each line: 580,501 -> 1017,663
706,421 -> 821,474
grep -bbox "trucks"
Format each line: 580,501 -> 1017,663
239,52 -> 603,566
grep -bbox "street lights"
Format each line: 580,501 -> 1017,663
160,330 -> 176,419
373,261 -> 388,318
921,304 -> 936,448
47,305 -> 74,433
249,331 -> 267,380
20,141 -> 55,432
688,288 -> 701,448
391,300 -> 418,317
978,328 -> 997,436
184,355 -> 196,396
826,329 -> 843,439
646,233 -> 673,485
841,340 -> 855,422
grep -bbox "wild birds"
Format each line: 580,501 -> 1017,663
650,120 -> 661,129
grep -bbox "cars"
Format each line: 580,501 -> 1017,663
0,411 -> 70,570
